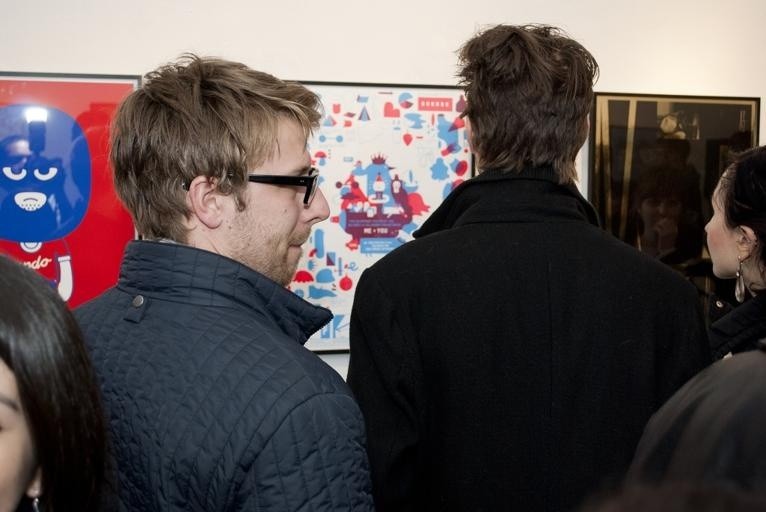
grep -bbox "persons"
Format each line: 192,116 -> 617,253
623,131 -> 752,265
346,23 -> 708,511
704,147 -> 766,356
1,256 -> 120,511
621,340 -> 766,512
68,53 -> 377,510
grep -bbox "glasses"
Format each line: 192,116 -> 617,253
248,168 -> 320,202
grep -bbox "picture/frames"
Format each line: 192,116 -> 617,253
279,79 -> 476,356
585,90 -> 762,334
0,70 -> 144,308
